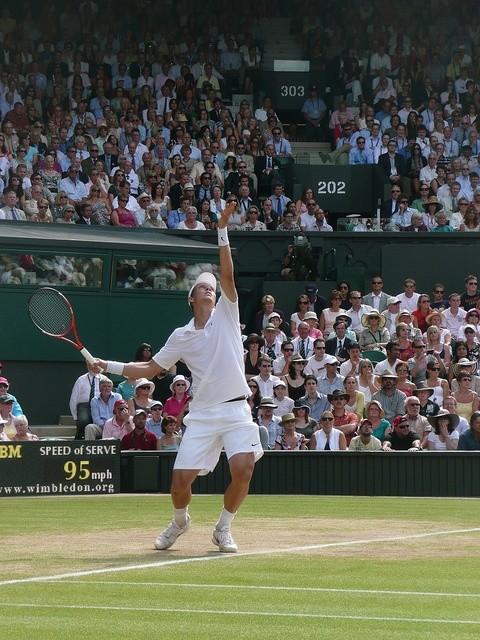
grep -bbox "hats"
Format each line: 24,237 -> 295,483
134,377 -> 155,396
261,323 -> 281,336
425,311 -> 447,327
305,286 -> 318,295
336,313 -> 352,326
393,414 -> 408,431
0,394 -> 15,404
360,308 -> 387,328
133,408 -> 147,417
169,374 -> 190,393
302,311 -> 319,322
0,413 -> 9,424
174,114 -> 188,122
255,396 -> 278,409
463,308 -> 480,324
0,377 -> 9,386
377,369 -> 401,386
224,151 -> 237,161
292,399 -> 311,413
396,309 -> 414,324
273,380 -> 286,388
364,399 -> 385,418
422,195 -> 443,211
308,84 -> 318,92
412,382 -> 434,397
452,358 -> 478,377
327,389 -> 350,403
137,191 -> 152,203
427,408 -> 460,430
278,413 -> 300,427
113,400 -> 129,414
150,400 -> 163,411
387,296 -> 402,307
267,312 -> 283,325
323,355 -> 340,366
456,44 -> 465,51
184,183 -> 194,191
187,271 -> 217,309
359,418 -> 373,429
464,324 -> 476,335
243,332 -> 265,351
288,354 -> 309,369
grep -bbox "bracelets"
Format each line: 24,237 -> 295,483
444,435 -> 450,438
106,359 -> 124,376
217,226 -> 229,247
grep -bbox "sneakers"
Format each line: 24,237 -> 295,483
211,522 -> 237,553
153,513 -> 192,550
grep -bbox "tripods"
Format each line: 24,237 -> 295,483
290,250 -> 314,283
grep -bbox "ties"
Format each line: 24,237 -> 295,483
126,176 -> 129,180
276,197 -> 282,214
79,151 -> 83,160
163,98 -> 167,117
267,347 -> 276,359
131,154 -> 136,171
268,158 -> 272,168
213,156 -> 217,164
338,341 -> 343,356
218,111 -> 220,116
299,340 -> 305,359
10,209 -> 17,219
462,130 -> 466,142
89,376 -> 95,401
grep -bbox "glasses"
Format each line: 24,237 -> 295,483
469,282 -> 477,285
414,345 -> 425,349
178,167 -> 185,169
177,129 -> 182,131
462,168 -> 469,171
154,407 -> 162,411
70,150 -> 77,153
298,301 -> 308,305
399,424 -> 409,429
143,350 -> 151,352
422,300 -> 429,304
351,296 -> 362,300
344,128 -> 351,131
358,142 -> 365,144
204,176 -> 211,180
369,316 -> 379,320
410,403 -> 421,406
405,100 -> 412,103
250,212 -> 257,214
308,203 -> 316,206
463,377 -> 472,381
270,119 -> 277,122
465,330 -> 474,334
34,178 -> 42,180
322,417 -> 333,421
20,150 -> 26,152
133,119 -> 138,122
90,149 -> 98,152
120,406 -> 128,410
362,424 -> 372,428
93,189 -> 100,192
121,199 -> 128,202
339,319 -> 348,322
61,195 -> 67,199
367,119 -> 373,122
430,367 -> 440,371
462,122 -> 468,125
117,173 -> 124,176
469,313 -> 479,317
297,361 -> 305,365
0,385 -> 7,388
67,210 -> 75,213
141,386 -> 150,390
420,188 -> 429,191
37,126 -> 43,128
316,346 -> 325,350
339,286 -> 347,290
372,282 -> 382,284
78,128 -> 83,130
212,147 -> 219,150
237,147 -> 244,150
333,396 -> 344,401
249,385 -> 257,388
284,348 -> 294,352
274,133 -> 281,135
228,199 -> 238,204
436,291 -> 444,294
158,129 -> 163,133
460,203 -> 468,206
177,384 -> 186,386
334,298 -> 341,300
39,205 -> 48,209
391,190 -> 400,193
238,166 -> 246,169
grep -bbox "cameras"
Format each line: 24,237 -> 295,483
424,348 -> 435,356
285,231 -> 318,288
